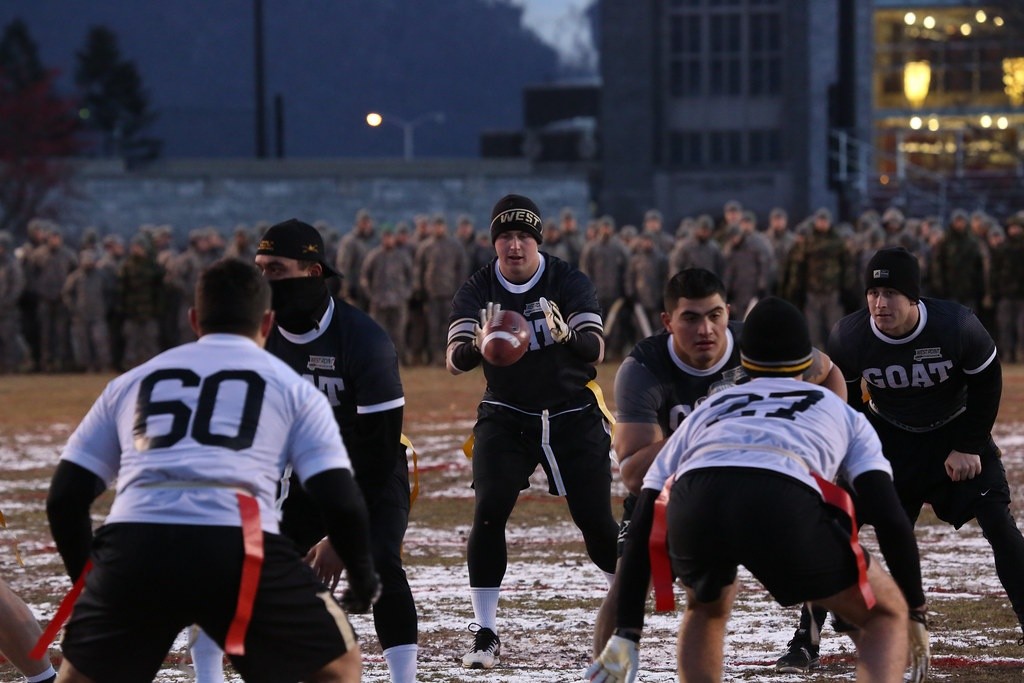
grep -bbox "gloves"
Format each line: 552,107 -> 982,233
906,611 -> 930,683
584,628 -> 641,683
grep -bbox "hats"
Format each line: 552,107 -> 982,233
256,219 -> 341,274
740,299 -> 814,375
490,193 -> 546,245
866,249 -> 926,303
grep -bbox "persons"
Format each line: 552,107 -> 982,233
185,217 -> 419,683
772,244 -> 1024,674
0,199 -> 1024,378
446,193 -> 625,669
584,266 -> 932,683
47,256 -> 367,683
0,576 -> 58,683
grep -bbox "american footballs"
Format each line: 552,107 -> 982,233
477,310 -> 532,366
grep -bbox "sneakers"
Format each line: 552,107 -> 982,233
775,628 -> 821,674
461,623 -> 501,669
